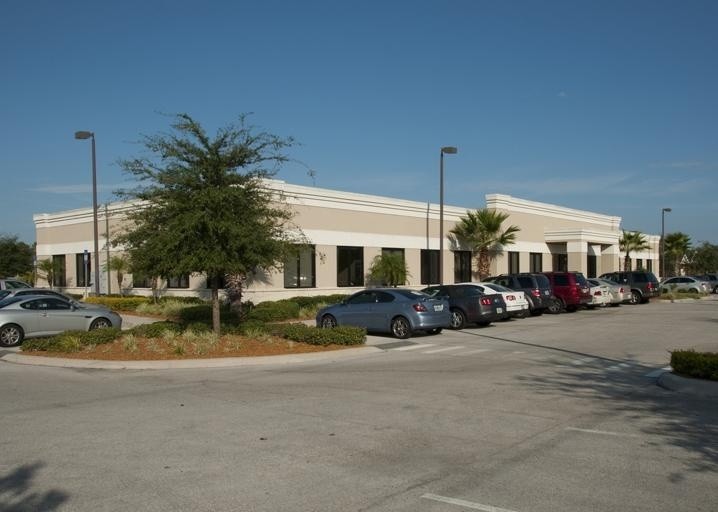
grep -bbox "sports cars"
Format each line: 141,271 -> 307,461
0,295 -> 122,347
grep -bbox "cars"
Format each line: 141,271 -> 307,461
586,279 -> 609,309
699,273 -> 718,294
0,288 -> 83,305
659,276 -> 712,296
0,279 -> 33,291
458,282 -> 529,320
315,287 -> 450,338
593,278 -> 633,307
420,284 -> 509,330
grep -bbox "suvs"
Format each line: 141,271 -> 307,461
534,271 -> 590,312
598,270 -> 660,304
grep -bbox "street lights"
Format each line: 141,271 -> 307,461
661,207 -> 672,280
73,130 -> 100,295
439,146 -> 458,285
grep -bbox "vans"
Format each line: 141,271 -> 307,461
481,272 -> 554,318
